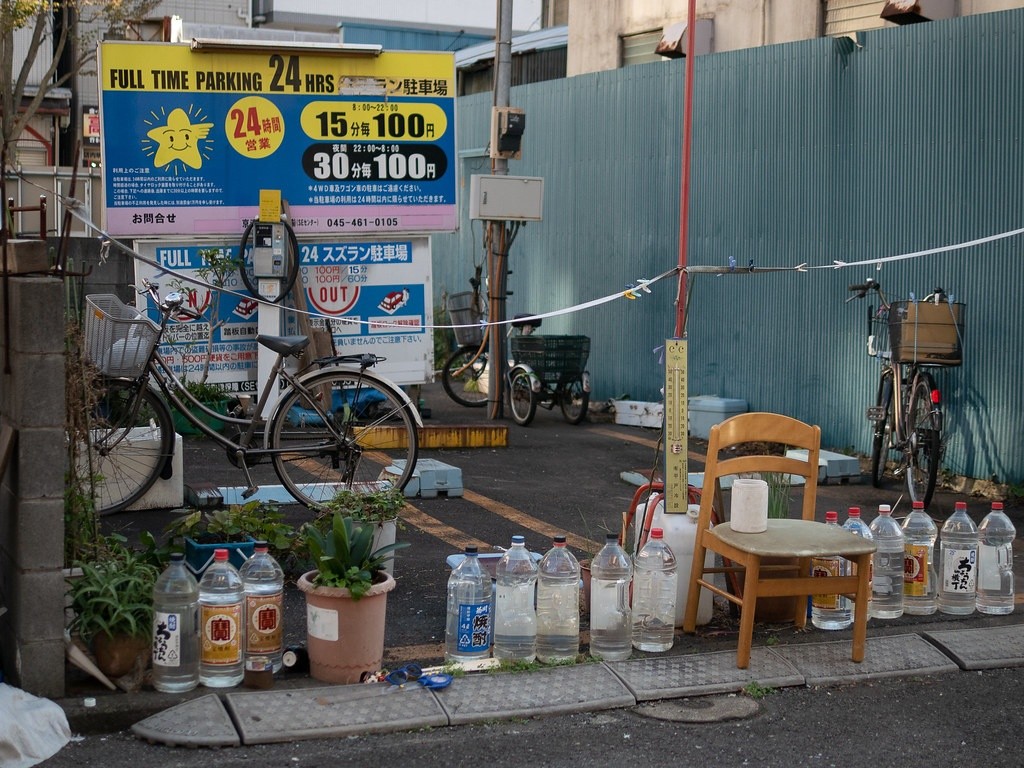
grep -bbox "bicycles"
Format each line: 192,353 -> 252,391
847,276 -> 966,512
68,277 -> 423,517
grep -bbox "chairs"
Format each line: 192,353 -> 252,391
682,411 -> 872,666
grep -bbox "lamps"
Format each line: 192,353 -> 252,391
189,37 -> 384,58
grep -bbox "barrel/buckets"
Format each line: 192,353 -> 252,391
634,493 -> 715,626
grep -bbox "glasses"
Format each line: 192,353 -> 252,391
384,664 -> 422,684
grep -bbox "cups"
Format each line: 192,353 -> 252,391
730,479 -> 769,533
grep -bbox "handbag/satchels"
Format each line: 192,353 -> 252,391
103,315 -> 148,377
889,292 -> 966,365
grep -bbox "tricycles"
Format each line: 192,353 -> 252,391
441,265 -> 591,427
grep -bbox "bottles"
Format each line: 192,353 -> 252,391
536,535 -> 581,665
493,535 -> 540,665
152,553 -> 200,695
810,510 -> 852,630
842,507 -> 874,623
868,504 -> 904,620
238,541 -> 285,682
937,501 -> 980,616
900,502 -> 939,616
198,549 -> 247,689
976,502 -> 1017,615
245,656 -> 273,690
589,532 -> 634,662
632,528 -> 679,652
443,545 -> 493,665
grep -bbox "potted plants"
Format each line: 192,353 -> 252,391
304,506 -> 398,680
723,449 -> 811,624
327,486 -> 403,582
78,547 -> 160,678
252,500 -> 298,576
178,506 -> 260,575
161,246 -> 247,436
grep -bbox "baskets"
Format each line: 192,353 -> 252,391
83,294 -> 163,378
867,304 -> 892,360
446,290 -> 483,344
